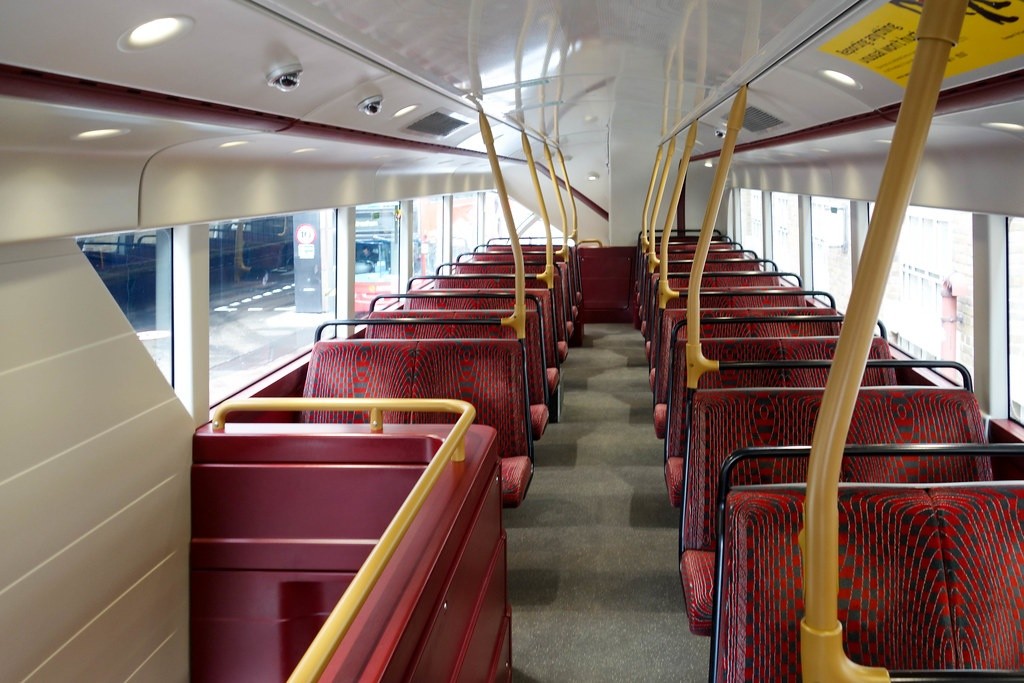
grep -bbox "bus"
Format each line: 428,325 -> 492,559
351,199 -> 434,315
76,212 -> 294,307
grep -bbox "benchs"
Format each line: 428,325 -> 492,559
636,229 -> 1024,683
302,234 -> 581,510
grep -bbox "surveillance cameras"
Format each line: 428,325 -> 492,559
265,62 -> 304,92
358,96 -> 383,115
715,128 -> 727,140
